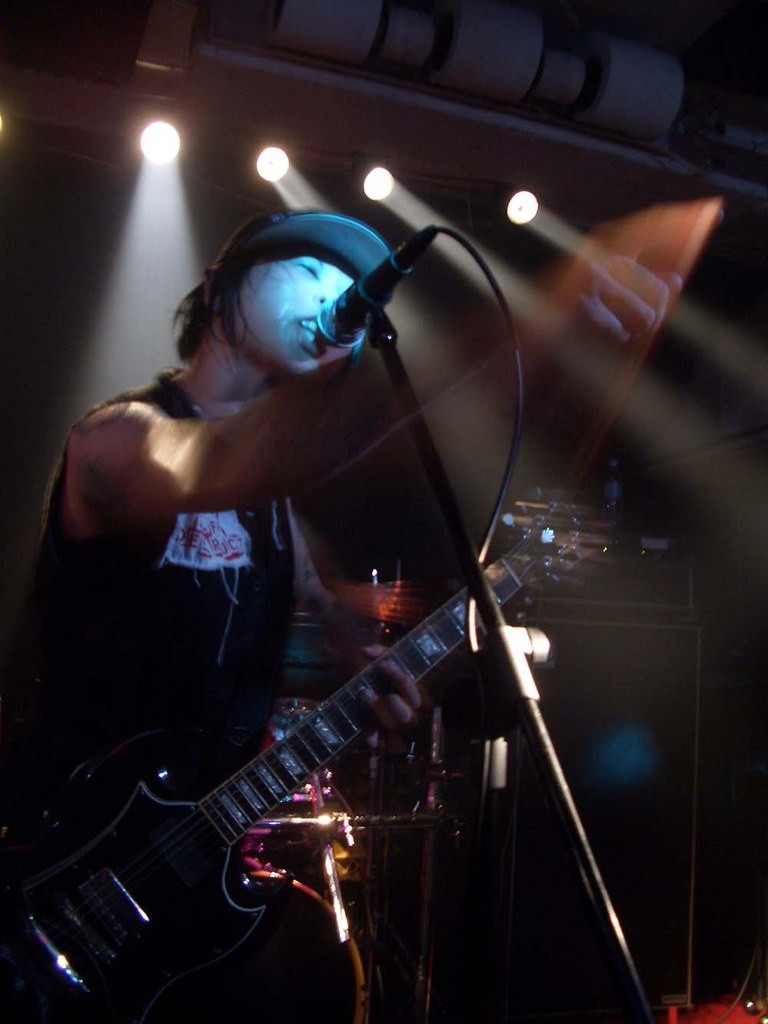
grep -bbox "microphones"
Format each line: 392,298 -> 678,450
317,226 -> 440,348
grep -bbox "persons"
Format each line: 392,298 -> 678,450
0,207 -> 435,1024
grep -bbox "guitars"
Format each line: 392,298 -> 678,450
0,504 -> 585,1023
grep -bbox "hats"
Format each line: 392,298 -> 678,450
210,209 -> 392,284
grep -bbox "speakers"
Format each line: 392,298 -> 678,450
456,615 -> 703,1024
